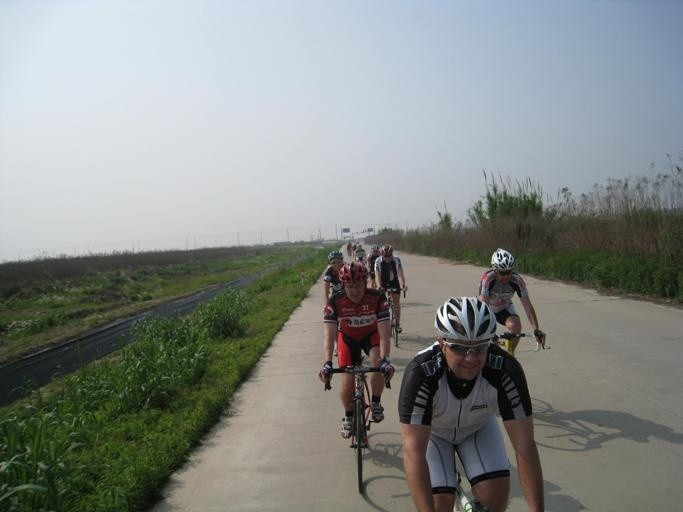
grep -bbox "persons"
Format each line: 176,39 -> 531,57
397,297 -> 545,512
476,247 -> 545,357
318,238 -> 407,440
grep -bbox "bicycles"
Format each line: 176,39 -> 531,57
354,258 -> 367,268
380,284 -> 408,347
321,360 -> 393,494
491,327 -> 553,359
448,469 -> 492,511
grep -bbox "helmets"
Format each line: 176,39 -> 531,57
338,261 -> 369,284
347,241 -> 378,258
490,247 -> 515,272
379,244 -> 394,257
328,251 -> 344,262
434,296 -> 497,342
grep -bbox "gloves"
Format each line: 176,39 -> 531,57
318,361 -> 333,384
379,356 -> 395,384
534,329 -> 545,346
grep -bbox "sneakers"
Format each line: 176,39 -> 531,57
340,416 -> 353,439
370,401 -> 384,424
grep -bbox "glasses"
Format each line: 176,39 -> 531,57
344,283 -> 364,289
444,339 -> 492,355
498,270 -> 512,275
